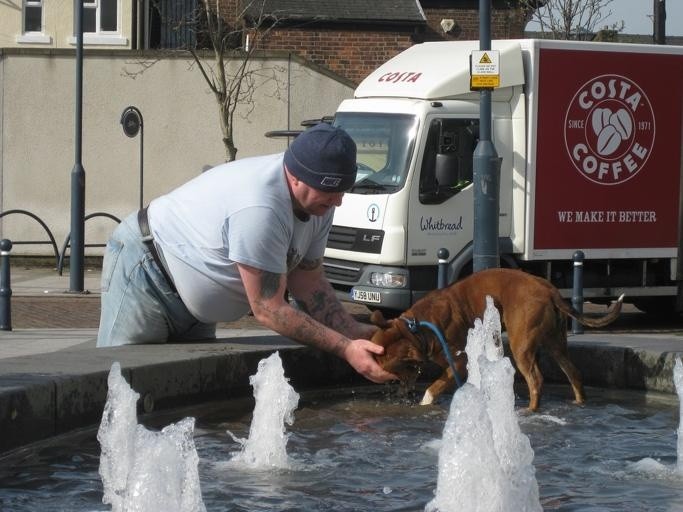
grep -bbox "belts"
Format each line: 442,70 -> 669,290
137,206 -> 177,296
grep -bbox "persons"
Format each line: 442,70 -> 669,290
94,122 -> 402,385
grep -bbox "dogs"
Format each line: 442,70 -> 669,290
368,265 -> 628,416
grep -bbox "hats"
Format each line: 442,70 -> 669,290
284,122 -> 357,194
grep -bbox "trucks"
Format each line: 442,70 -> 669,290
324,37 -> 682,326
